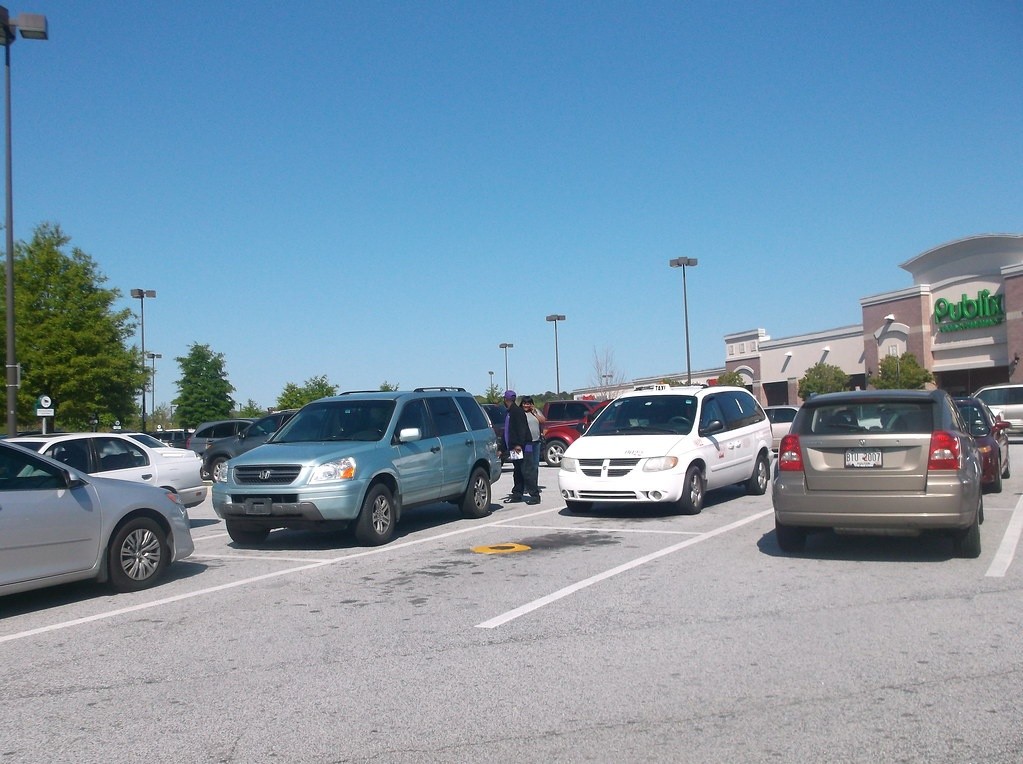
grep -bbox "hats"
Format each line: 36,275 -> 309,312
503,390 -> 516,399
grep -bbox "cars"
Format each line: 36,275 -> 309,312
773,391 -> 986,560
879,398 -> 1013,496
0,441 -> 195,605
756,406 -> 804,453
1,432 -> 210,519
556,385 -> 774,514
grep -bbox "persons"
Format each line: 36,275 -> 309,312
519,396 -> 545,467
497,390 -> 540,505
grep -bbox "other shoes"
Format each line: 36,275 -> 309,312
526,497 -> 540,505
537,486 -> 542,492
512,486 -> 515,493
504,497 -> 522,503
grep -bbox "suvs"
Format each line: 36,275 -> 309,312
212,385 -> 503,547
147,429 -> 191,449
542,399 -> 630,467
538,400 -> 619,434
186,417 -> 272,458
198,407 -> 344,484
481,404 -> 512,467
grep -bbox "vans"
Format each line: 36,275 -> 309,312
956,383 -> 1023,437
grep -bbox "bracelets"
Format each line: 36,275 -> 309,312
533,412 -> 537,415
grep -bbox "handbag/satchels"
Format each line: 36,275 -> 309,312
540,435 -> 545,445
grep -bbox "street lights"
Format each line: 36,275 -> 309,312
0,7 -> 50,446
499,343 -> 513,394
488,371 -> 494,395
147,353 -> 163,418
546,315 -> 566,399
170,405 -> 177,424
669,255 -> 699,384
129,287 -> 156,436
